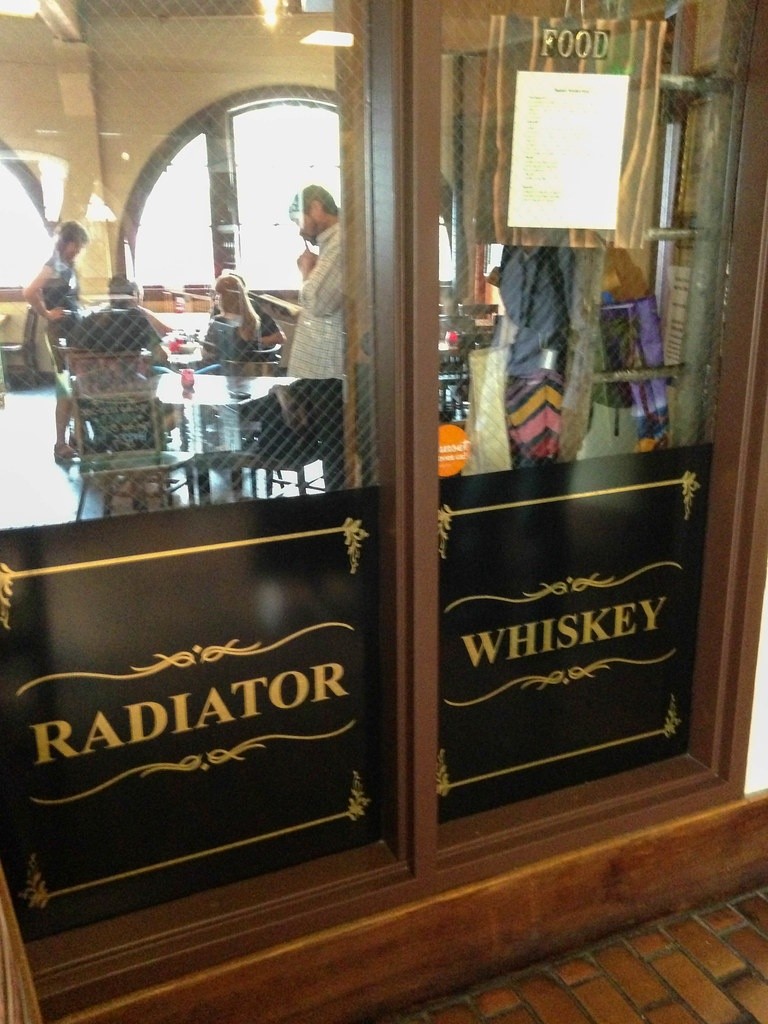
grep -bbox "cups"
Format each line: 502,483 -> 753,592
181,369 -> 194,389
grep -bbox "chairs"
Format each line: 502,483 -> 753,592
0,305 -> 40,393
67,391 -> 194,522
437,300 -> 505,431
55,342 -> 181,476
225,377 -> 328,499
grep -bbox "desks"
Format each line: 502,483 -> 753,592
145,371 -> 302,500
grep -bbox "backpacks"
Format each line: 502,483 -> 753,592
262,385 -> 317,455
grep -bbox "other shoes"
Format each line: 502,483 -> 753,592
54,440 -> 79,459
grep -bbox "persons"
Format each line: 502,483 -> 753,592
201,273 -> 287,362
22,219 -> 91,461
262,185 -> 346,493
476,154 -> 661,468
67,275 -> 183,445
